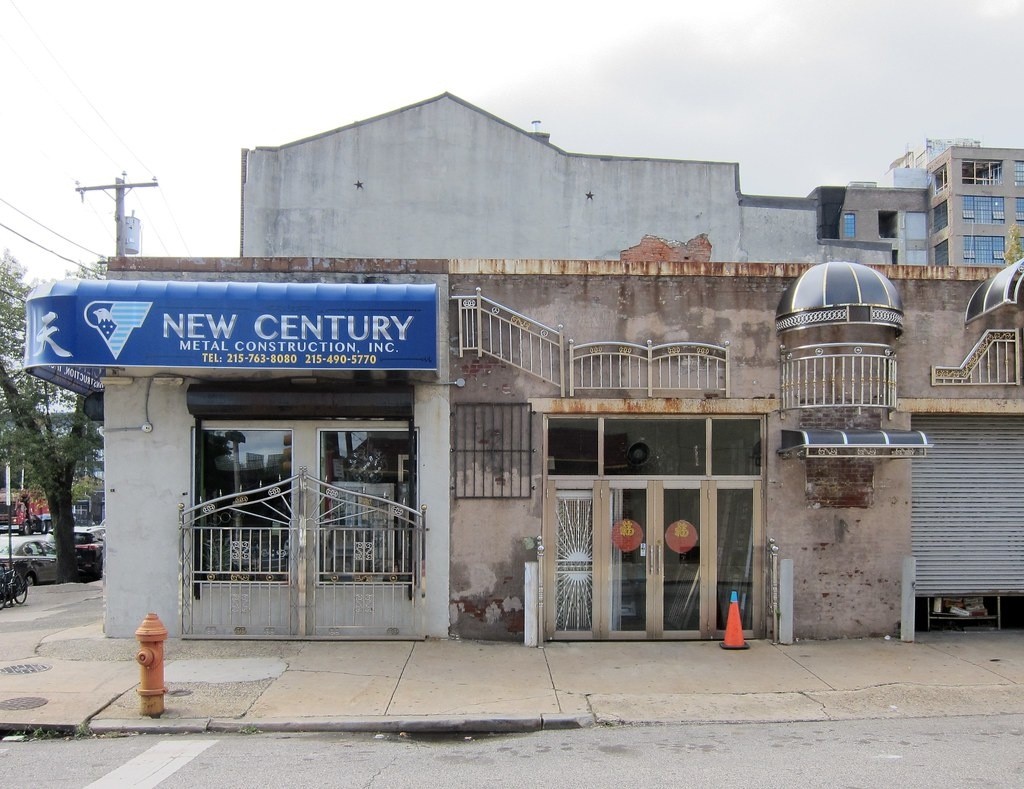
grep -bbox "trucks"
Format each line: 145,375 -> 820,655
0,501 -> 52,535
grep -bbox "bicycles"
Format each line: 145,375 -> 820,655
0,563 -> 28,610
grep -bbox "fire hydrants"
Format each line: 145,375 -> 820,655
134,612 -> 168,716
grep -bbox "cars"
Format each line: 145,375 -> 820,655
0,519 -> 105,586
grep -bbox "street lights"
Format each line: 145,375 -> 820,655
225,431 -> 246,541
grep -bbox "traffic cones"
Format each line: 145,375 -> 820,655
719,591 -> 751,649
